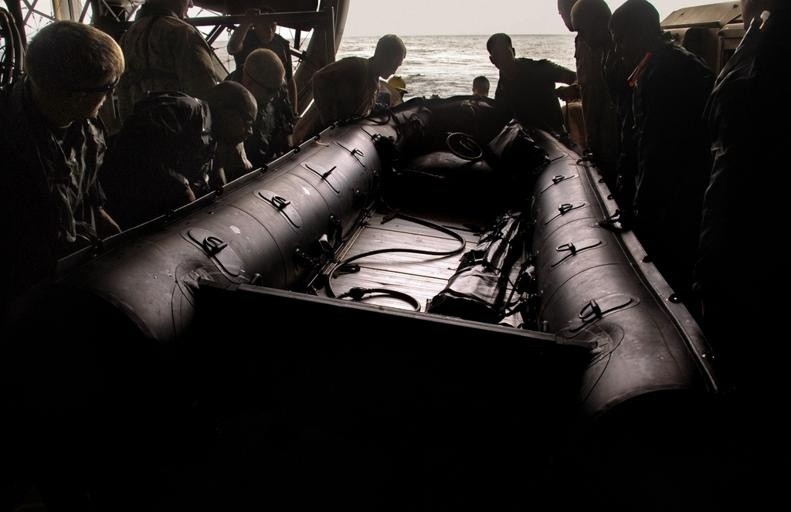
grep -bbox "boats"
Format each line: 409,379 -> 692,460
51,79 -> 740,461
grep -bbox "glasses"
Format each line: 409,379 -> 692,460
241,71 -> 288,96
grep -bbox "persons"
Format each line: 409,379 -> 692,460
468,0 -> 790,359
0,0 -> 410,287
